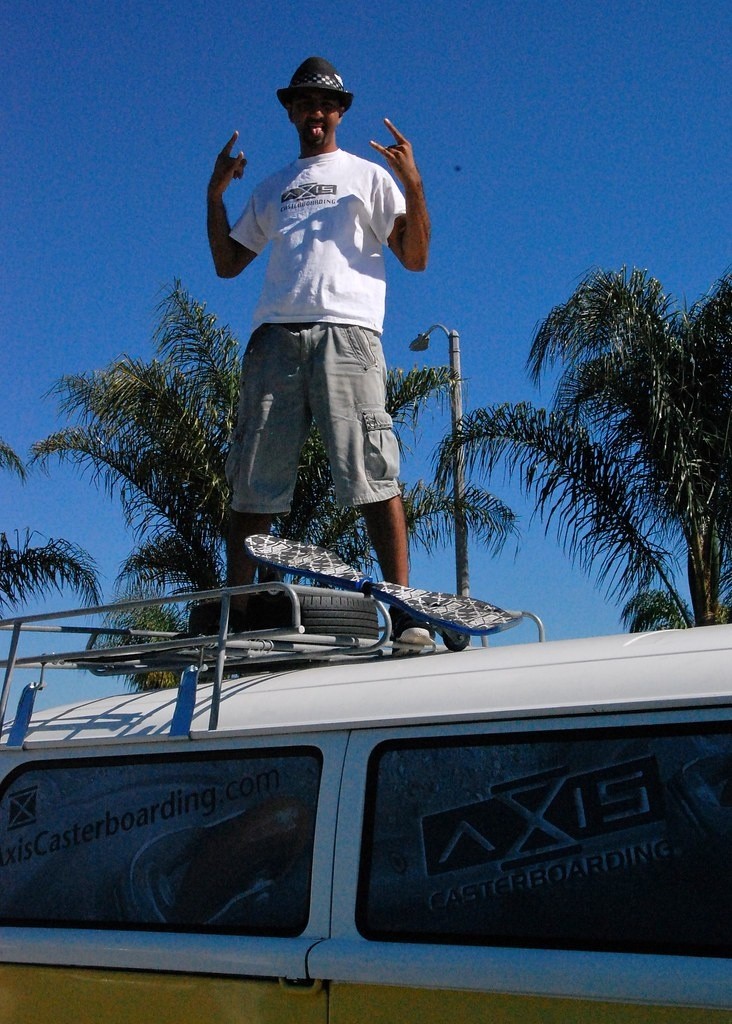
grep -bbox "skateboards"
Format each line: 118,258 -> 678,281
247,534 -> 522,654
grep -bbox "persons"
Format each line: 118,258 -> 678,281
206,56 -> 435,658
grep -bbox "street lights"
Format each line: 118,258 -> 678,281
409,324 -> 469,598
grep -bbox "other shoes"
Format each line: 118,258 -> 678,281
387,608 -> 435,657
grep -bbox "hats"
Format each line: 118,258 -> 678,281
277,57 -> 354,112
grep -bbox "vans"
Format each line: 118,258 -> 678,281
0,585 -> 732,1024
